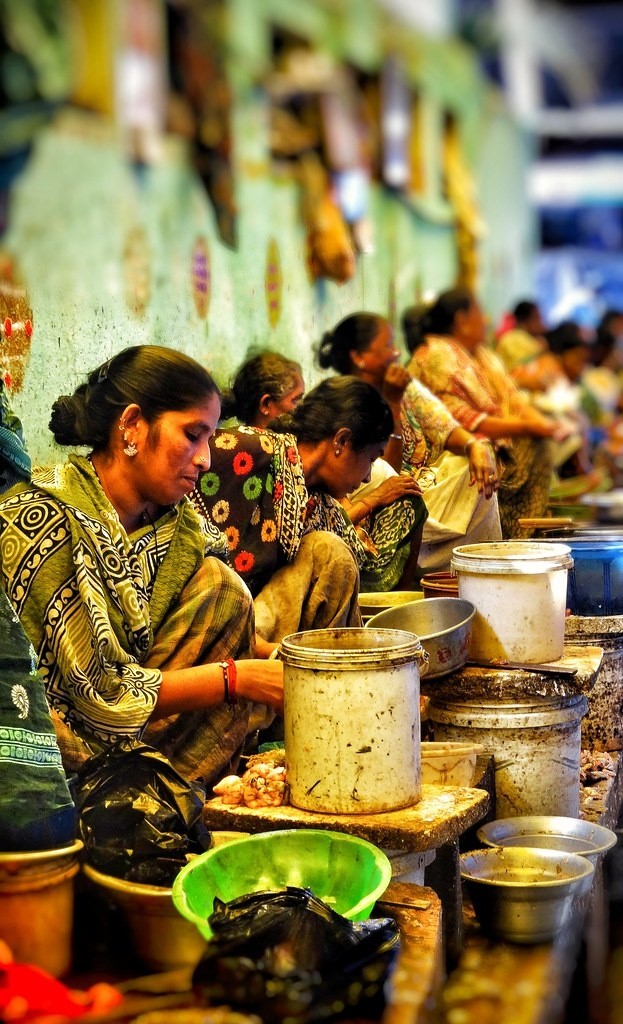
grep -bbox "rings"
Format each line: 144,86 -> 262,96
489,476 -> 494,479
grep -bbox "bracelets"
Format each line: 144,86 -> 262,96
221,660 -> 236,702
464,439 -> 475,453
360,498 -> 373,509
391,434 -> 403,440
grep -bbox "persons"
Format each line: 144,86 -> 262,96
0,286 -> 623,799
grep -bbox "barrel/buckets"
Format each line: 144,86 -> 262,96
80,832 -> 254,970
278,627 -> 430,815
420,571 -> 459,598
0,839 -> 83,982
449,543 -> 576,664
430,668 -> 590,822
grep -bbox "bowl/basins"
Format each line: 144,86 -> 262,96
511,537 -> 623,616
419,743 -> 484,787
170,828 -> 391,944
357,590 -> 423,616
477,816 -> 618,902
460,848 -> 593,944
367,596 -> 476,680
